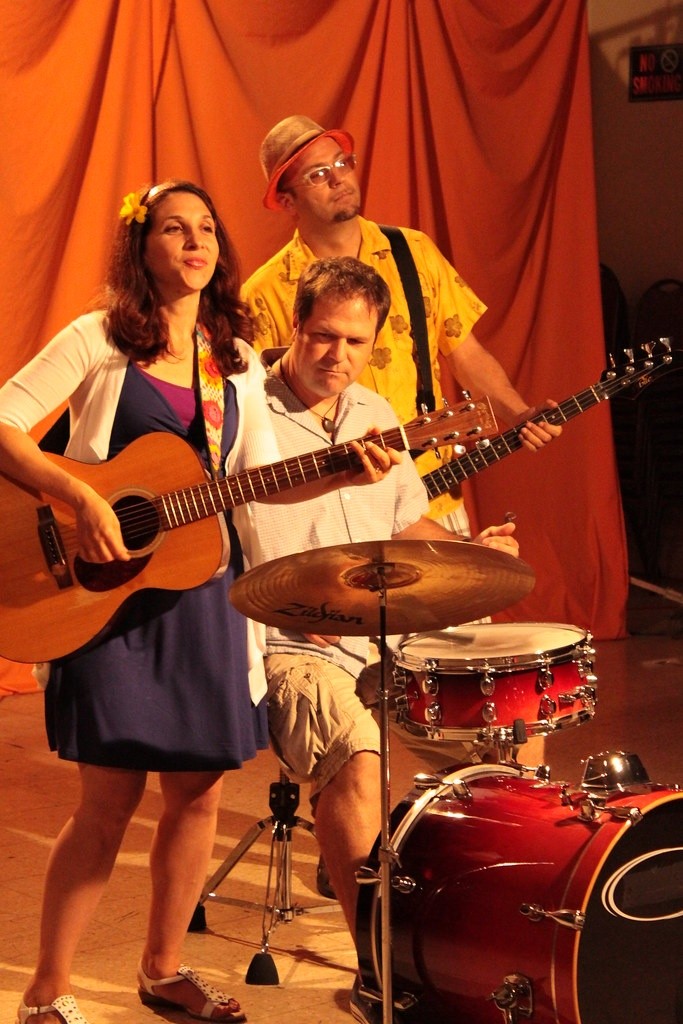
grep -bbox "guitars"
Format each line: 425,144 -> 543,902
411,336 -> 682,522
0,393 -> 501,666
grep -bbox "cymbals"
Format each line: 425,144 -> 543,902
225,537 -> 538,637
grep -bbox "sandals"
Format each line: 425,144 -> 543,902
18,992 -> 90,1024
137,954 -> 247,1024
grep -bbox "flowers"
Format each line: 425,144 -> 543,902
119,193 -> 149,225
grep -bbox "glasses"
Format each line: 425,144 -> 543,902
278,154 -> 359,186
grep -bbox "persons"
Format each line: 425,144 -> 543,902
232,115 -> 571,1022
0,172 -> 406,1024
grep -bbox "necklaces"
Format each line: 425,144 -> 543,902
280,351 -> 343,434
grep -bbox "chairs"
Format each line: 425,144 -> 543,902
599,264 -> 683,595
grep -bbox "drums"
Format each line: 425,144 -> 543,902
390,621 -> 600,746
352,748 -> 682,1024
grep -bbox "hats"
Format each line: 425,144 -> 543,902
260,115 -> 354,210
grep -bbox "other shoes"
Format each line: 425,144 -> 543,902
316,853 -> 337,899
349,978 -> 372,1024
